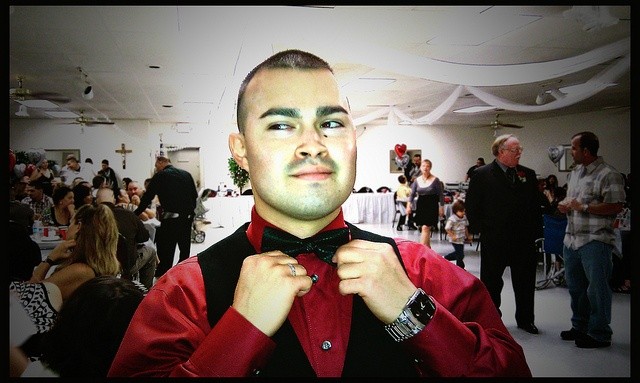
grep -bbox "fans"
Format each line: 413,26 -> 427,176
469,105 -> 523,135
57,109 -> 115,135
8,74 -> 69,109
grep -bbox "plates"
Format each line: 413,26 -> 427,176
42,236 -> 60,241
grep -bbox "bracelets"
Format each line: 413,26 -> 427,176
583,204 -> 588,212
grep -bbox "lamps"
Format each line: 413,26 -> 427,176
81,78 -> 94,102
14,104 -> 31,117
536,90 -> 547,106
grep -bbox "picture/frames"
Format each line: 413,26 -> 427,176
565,148 -> 576,171
389,149 -> 421,174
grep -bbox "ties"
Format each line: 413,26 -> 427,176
507,167 -> 515,181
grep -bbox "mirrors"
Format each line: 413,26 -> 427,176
558,144 -> 577,173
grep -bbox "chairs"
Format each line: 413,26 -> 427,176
393,190 -> 401,227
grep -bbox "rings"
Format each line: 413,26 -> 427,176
288,263 -> 296,276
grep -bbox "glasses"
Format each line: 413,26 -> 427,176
501,145 -> 524,153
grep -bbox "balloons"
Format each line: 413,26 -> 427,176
395,144 -> 406,158
393,154 -> 410,168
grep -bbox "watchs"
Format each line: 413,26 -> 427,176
384,288 -> 436,343
43,255 -> 54,266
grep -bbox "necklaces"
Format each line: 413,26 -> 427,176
423,175 -> 430,183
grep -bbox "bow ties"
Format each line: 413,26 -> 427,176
260,225 -> 349,265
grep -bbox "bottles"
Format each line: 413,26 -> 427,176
32,220 -> 43,241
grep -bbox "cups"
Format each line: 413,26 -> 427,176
44,227 -> 48,238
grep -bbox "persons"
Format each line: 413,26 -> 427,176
537,175 -> 567,285
442,199 -> 473,269
406,159 -> 444,249
464,134 -> 544,335
30,204 -> 117,305
464,158 -> 486,182
557,131 -> 627,349
9,277 -> 148,381
397,175 -> 417,231
132,157 -> 198,278
4,149 -> 159,233
409,154 -> 420,231
106,50 -> 531,378
5,235 -> 63,377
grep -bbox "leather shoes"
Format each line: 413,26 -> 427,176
408,224 -> 417,229
575,334 -> 611,348
561,328 -> 587,339
518,321 -> 539,334
397,225 -> 402,230
